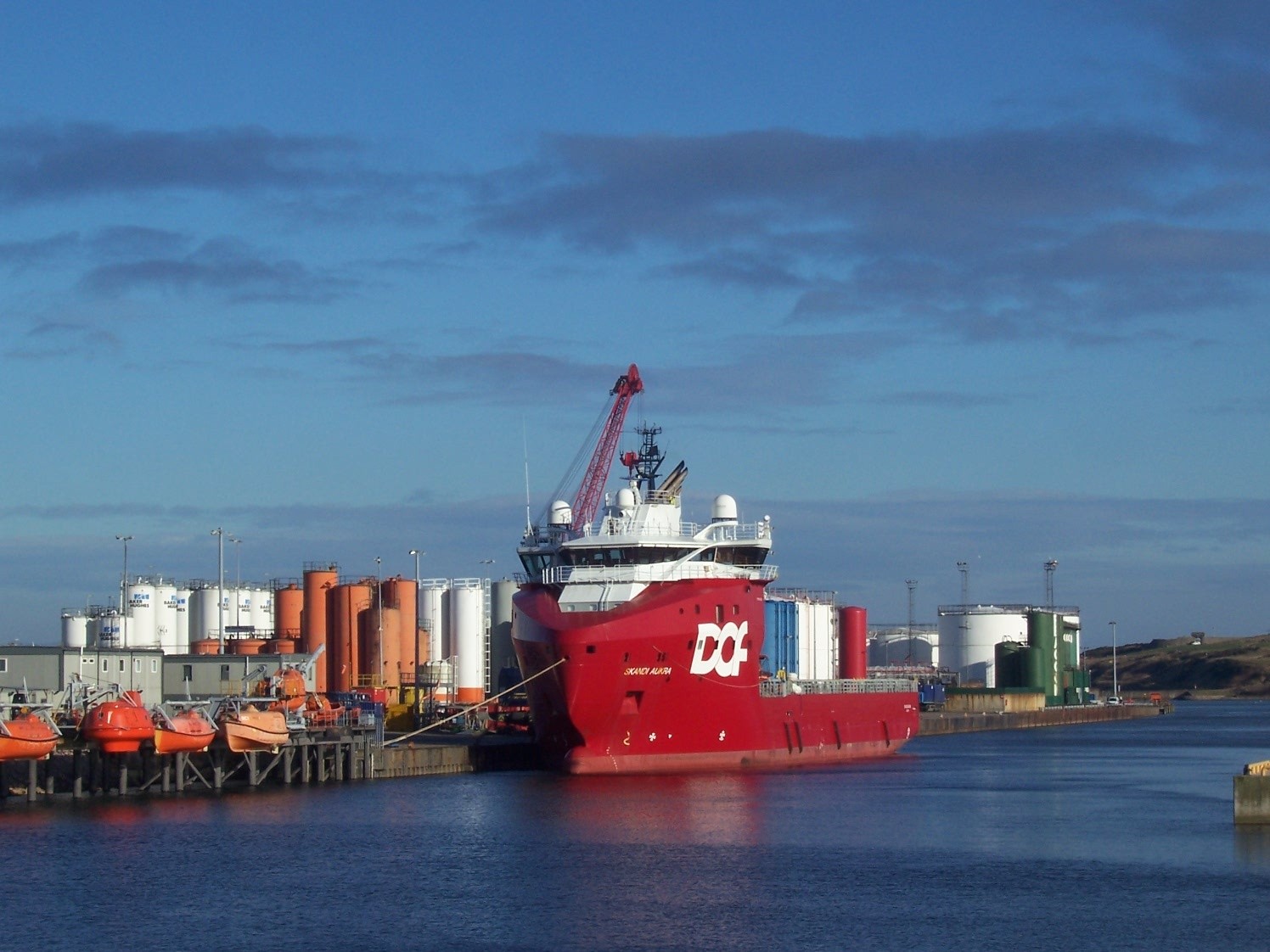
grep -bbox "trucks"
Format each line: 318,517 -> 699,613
1106,696 -> 1121,706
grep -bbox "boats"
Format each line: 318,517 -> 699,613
220,704 -> 290,756
255,667 -> 306,714
510,418 -> 922,774
154,710 -> 217,754
0,705 -> 60,760
301,691 -> 347,725
81,690 -> 155,752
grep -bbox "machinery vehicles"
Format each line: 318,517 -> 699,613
532,364 -> 646,538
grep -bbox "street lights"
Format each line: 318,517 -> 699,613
373,557 -> 383,686
409,549 -> 427,732
210,526 -> 233,653
1080,647 -> 1091,671
114,534 -> 133,649
1108,621 -> 1118,697
228,538 -> 244,639
